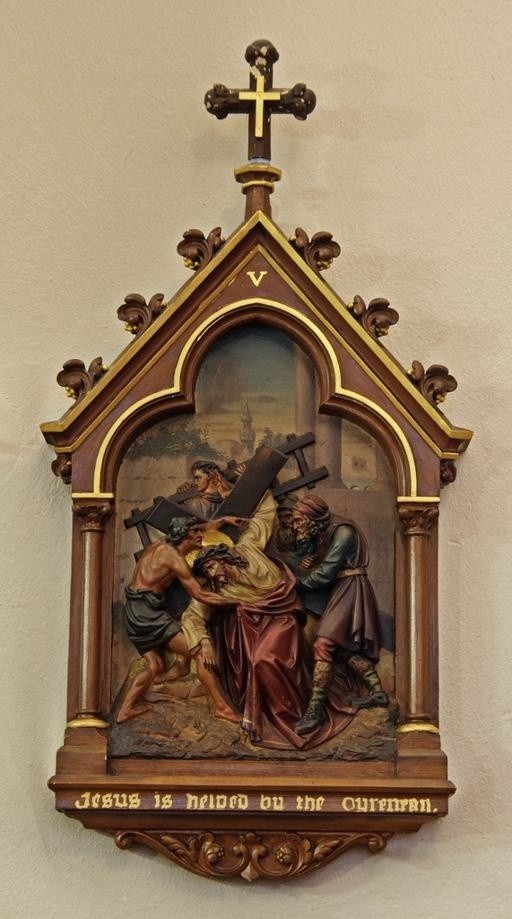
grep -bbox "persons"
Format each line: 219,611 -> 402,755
289,495 -> 390,736
110,513 -> 250,724
273,488 -> 330,652
153,460 -> 252,687
179,463 -> 365,751
